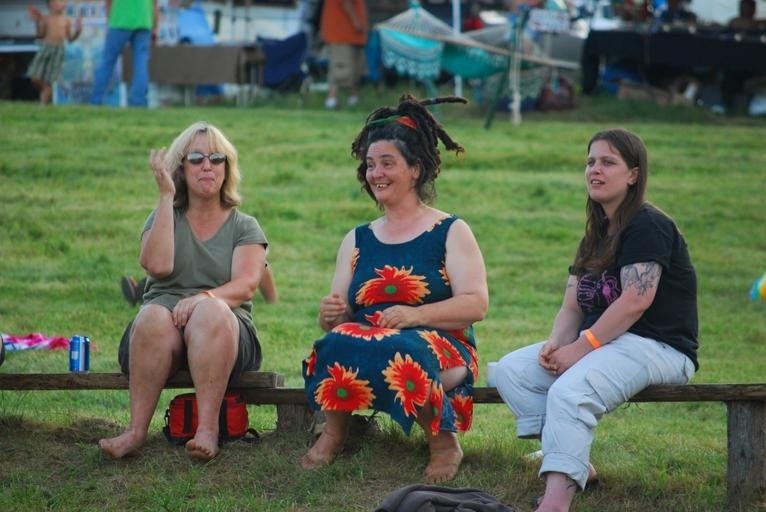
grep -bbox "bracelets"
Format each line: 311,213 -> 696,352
583,329 -> 601,349
200,291 -> 214,297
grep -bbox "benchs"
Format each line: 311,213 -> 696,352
1,369 -> 765,511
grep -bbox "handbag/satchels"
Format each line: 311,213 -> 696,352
162,390 -> 261,447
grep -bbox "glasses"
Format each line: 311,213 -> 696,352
184,151 -> 227,165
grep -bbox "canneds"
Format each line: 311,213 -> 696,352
70,336 -> 89,372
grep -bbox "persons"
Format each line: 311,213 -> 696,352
496,130 -> 701,511
88,1 -> 161,109
298,93 -> 490,484
173,1 -> 226,104
318,1 -> 368,110
719,0 -> 766,115
97,121 -> 268,461
26,1 -> 83,104
658,0 -> 699,24
